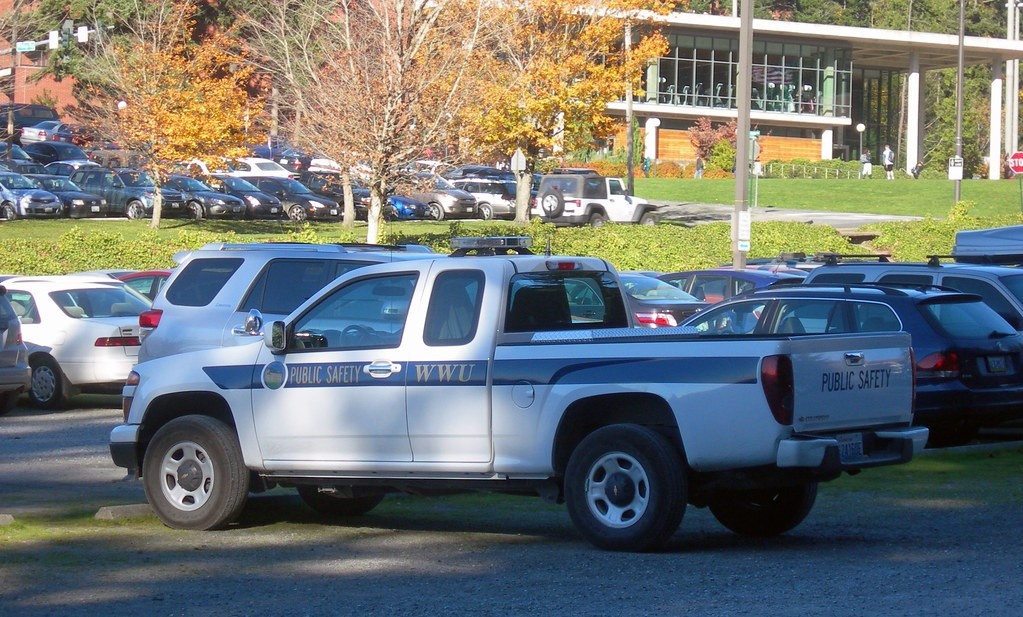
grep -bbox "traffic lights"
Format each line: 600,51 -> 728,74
62,27 -> 70,49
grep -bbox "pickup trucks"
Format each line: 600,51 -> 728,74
107,235 -> 929,554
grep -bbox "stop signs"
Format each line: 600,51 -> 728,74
1008,151 -> 1023,174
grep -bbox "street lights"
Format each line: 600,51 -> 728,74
855,123 -> 866,158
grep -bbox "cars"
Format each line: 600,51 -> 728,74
0,267 -> 176,412
0,101 -> 576,222
566,223 -> 1023,450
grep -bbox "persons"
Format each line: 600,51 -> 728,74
694,155 -> 703,179
863,147 -> 872,179
882,143 -> 894,180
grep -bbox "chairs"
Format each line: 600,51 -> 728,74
10,300 -> 34,324
509,285 -> 572,329
782,316 -> 805,334
63,306 -> 84,316
862,317 -> 885,331
430,280 -> 476,340
112,303 -> 135,316
828,308 -> 845,334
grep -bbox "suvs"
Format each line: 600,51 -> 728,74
133,243 -> 559,365
536,167 -> 660,231
